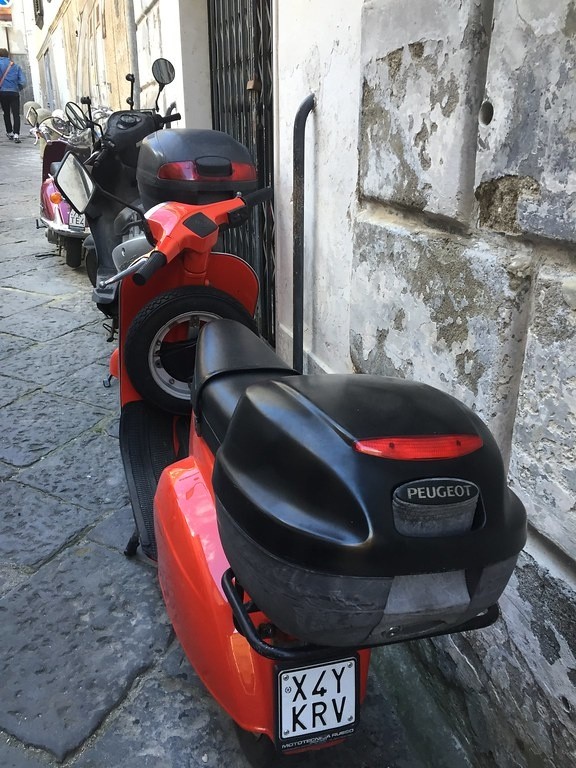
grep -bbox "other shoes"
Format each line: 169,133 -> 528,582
5,133 -> 14,140
12,133 -> 19,143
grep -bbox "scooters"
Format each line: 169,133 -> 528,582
52,143 -> 527,768
65,56 -> 257,343
25,103 -> 114,268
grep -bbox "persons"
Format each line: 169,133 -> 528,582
0,47 -> 29,143
